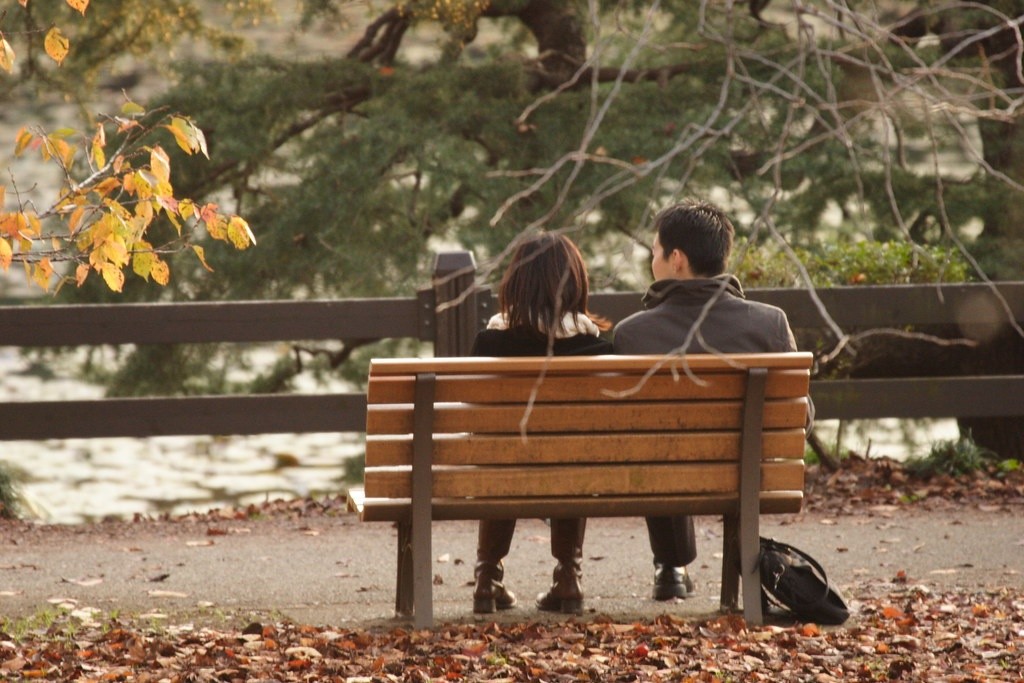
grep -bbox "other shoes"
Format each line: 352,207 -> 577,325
652,562 -> 692,600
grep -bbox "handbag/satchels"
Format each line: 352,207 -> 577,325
734,535 -> 850,626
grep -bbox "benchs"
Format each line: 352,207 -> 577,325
346,350 -> 815,631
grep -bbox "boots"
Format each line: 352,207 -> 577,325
536,516 -> 586,616
472,515 -> 515,613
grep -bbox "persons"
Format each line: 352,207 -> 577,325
613,200 -> 814,600
466,230 -> 614,616
